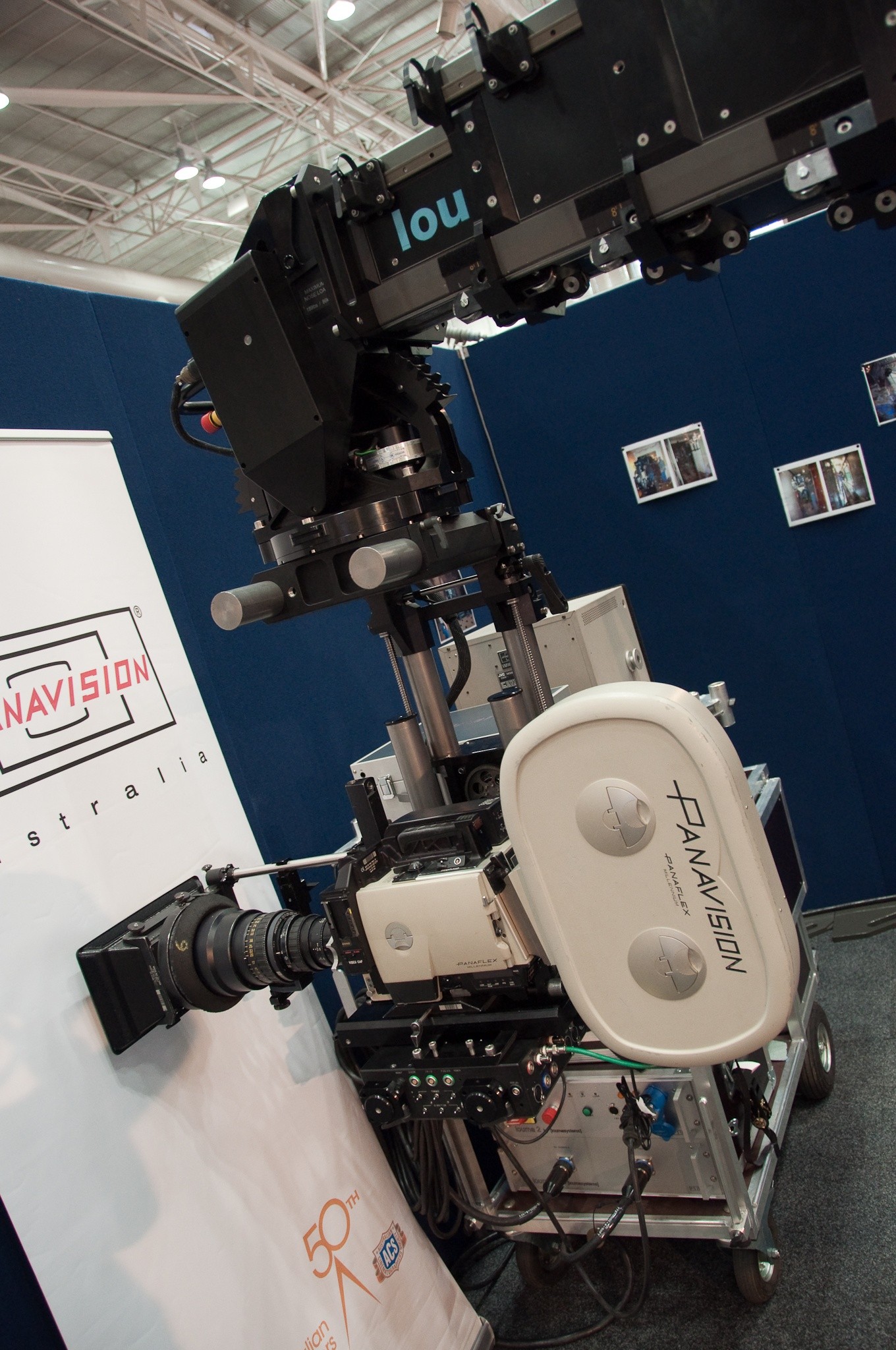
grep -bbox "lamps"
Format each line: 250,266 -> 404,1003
162,106 -> 226,190
327,0 -> 357,21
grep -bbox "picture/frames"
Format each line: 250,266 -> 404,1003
860,352 -> 896,427
621,422 -> 719,505
773,443 -> 877,528
